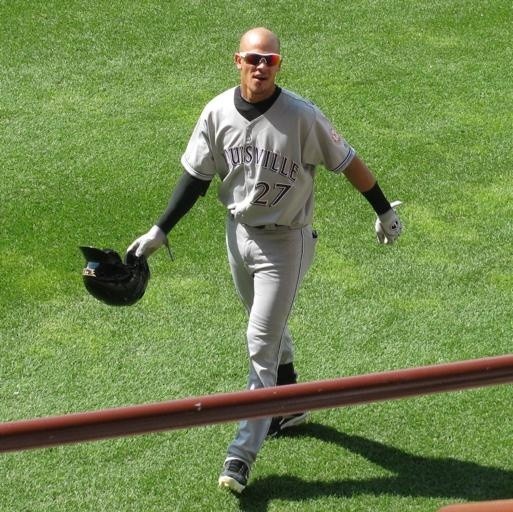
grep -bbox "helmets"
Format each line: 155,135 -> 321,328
77,246 -> 149,306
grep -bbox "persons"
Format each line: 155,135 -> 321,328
124,26 -> 405,496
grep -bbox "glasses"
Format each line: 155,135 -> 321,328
239,51 -> 282,66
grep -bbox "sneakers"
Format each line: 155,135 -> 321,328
219,459 -> 249,494
264,410 -> 307,440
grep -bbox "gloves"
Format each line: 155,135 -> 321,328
379,209 -> 402,244
127,224 -> 167,257
